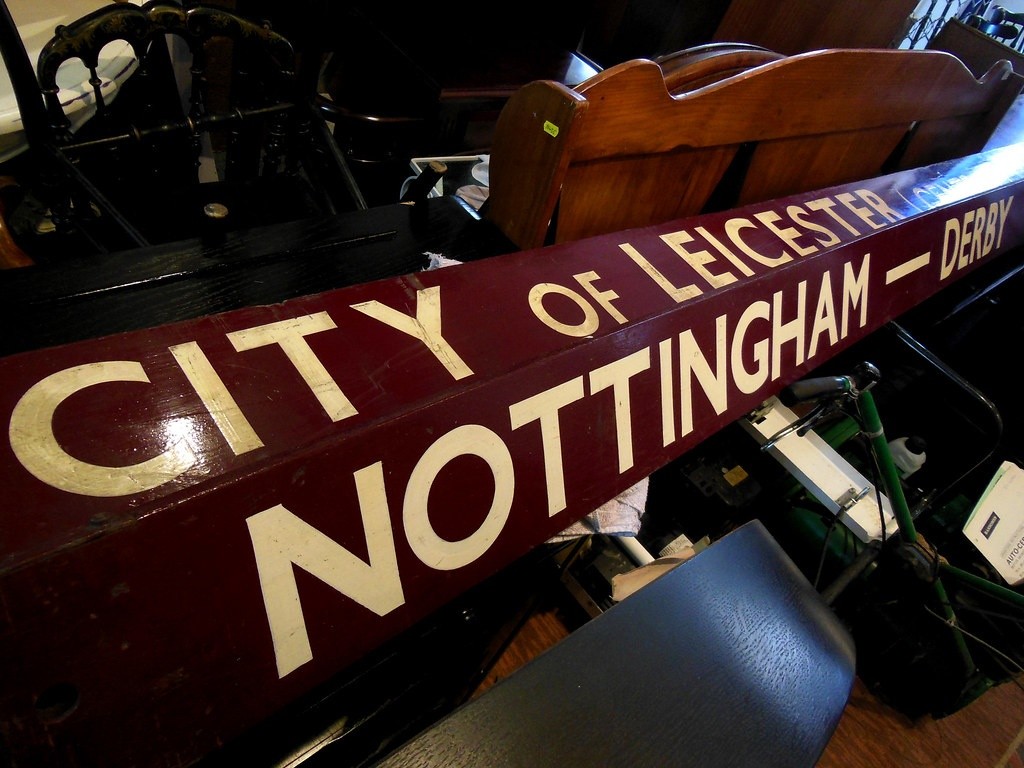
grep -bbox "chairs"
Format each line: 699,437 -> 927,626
481,37 -> 1024,255
35,2 -> 367,264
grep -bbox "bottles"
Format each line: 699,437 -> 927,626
889,436 -> 926,479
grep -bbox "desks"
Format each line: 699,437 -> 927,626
0,196 -> 522,358
